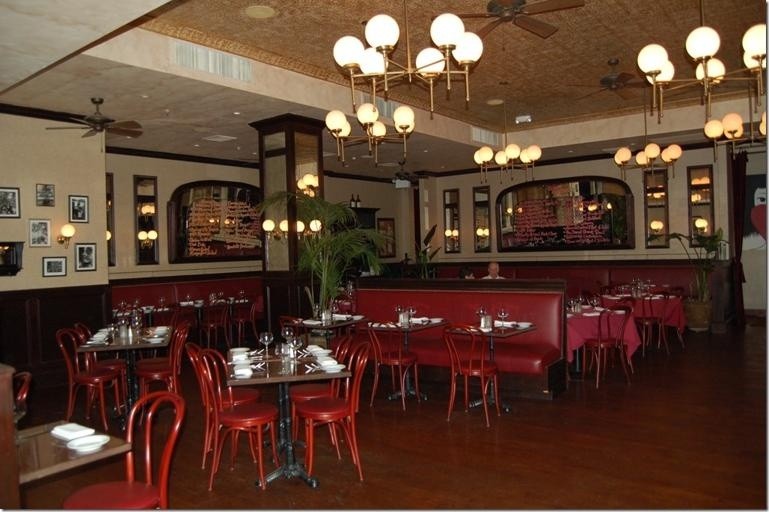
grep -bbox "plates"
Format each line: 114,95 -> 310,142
84,323 -> 171,345
409,315 -> 444,326
301,313 -> 363,326
228,345 -> 346,375
141,303 -> 156,310
66,434 -> 111,454
480,320 -> 536,332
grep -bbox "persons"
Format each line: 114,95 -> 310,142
479,263 -> 506,280
456,267 -> 476,280
72,201 -> 85,219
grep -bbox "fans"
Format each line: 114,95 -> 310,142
433,1 -> 585,41
42,99 -> 146,142
567,59 -> 650,104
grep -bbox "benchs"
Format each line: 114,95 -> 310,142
309,291 -> 562,403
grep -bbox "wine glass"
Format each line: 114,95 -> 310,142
497,304 -> 511,318
157,288 -> 248,307
475,304 -> 488,318
567,275 -> 657,312
312,298 -> 354,319
281,326 -> 302,365
259,330 -> 274,360
394,303 -> 417,327
107,296 -> 144,344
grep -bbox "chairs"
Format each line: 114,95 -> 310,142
55,297 -> 536,427
184,338 -> 373,492
563,273 -> 688,392
4,365 -> 185,509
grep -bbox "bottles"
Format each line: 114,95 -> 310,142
350,192 -> 361,208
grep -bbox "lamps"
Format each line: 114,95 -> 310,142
146,230 -> 157,250
310,220 -> 321,240
138,230 -> 147,248
330,0 -> 485,118
279,220 -> 287,237
57,225 -> 75,249
296,220 -> 304,238
611,85 -> 682,184
261,218 -> 281,241
702,83 -> 767,163
470,81 -> 543,190
324,81 -> 416,166
634,0 -> 768,126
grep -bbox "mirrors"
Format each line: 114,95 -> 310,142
132,176 -> 161,264
105,173 -> 116,267
292,132 -> 325,268
441,163 -> 715,255
259,129 -> 291,273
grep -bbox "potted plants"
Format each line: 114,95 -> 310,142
645,224 -> 735,334
258,192 -> 394,353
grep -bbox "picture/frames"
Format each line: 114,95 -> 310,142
42,256 -> 67,276
74,244 -> 99,273
67,194 -> 91,225
29,217 -> 52,249
1,186 -> 21,219
36,183 -> 57,207
